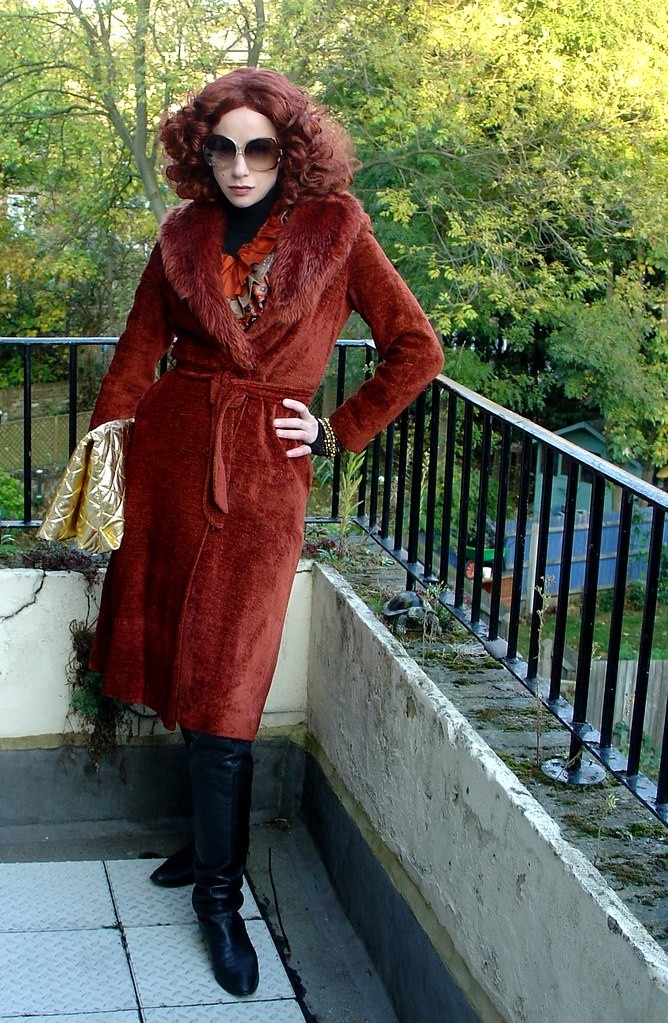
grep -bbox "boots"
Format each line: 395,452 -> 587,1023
149,727 -> 205,888
191,730 -> 260,995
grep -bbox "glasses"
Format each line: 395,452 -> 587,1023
199,133 -> 285,171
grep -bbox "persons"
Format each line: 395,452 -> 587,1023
82,66 -> 444,996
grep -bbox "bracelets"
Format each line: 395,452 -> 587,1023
318,418 -> 337,459
